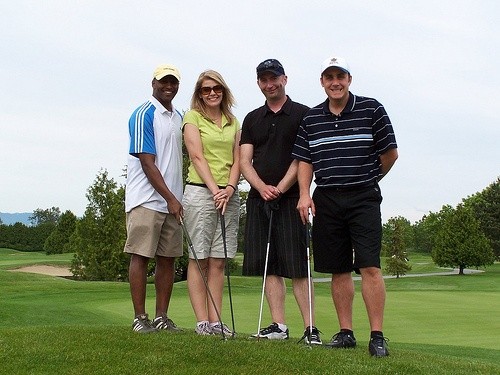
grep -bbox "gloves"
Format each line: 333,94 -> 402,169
264,192 -> 282,219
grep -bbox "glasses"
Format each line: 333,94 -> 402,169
199,85 -> 225,94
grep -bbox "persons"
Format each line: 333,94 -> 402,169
238,59 -> 322,344
122,65 -> 187,334
291,57 -> 398,356
180,70 -> 241,337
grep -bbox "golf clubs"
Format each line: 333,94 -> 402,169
256,210 -> 273,341
220,207 -> 237,339
179,214 -> 229,341
298,207 -> 312,351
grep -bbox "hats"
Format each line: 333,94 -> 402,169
320,57 -> 350,78
257,59 -> 285,79
154,64 -> 181,84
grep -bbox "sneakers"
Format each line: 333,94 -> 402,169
297,326 -> 324,345
249,323 -> 290,340
150,312 -> 181,332
324,331 -> 357,348
210,322 -> 237,337
369,335 -> 390,357
193,321 -> 216,335
132,314 -> 158,332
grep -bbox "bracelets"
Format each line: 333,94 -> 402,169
226,184 -> 236,191
